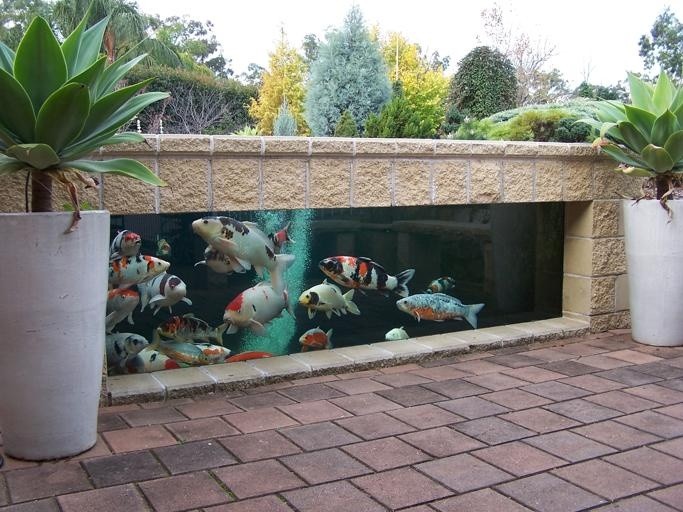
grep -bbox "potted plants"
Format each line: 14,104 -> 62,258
573,69 -> 682,350
0,0 -> 173,461
